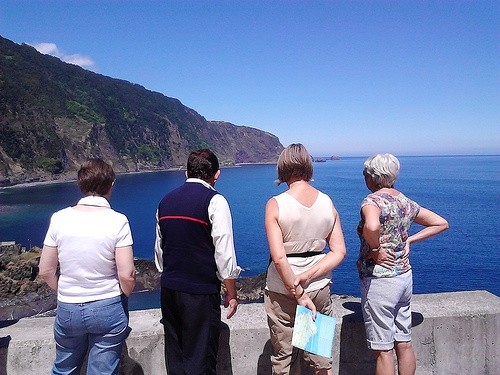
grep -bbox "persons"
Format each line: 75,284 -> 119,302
38,158 -> 137,375
356,152 -> 449,375
261,143 -> 347,375
153,149 -> 245,375
